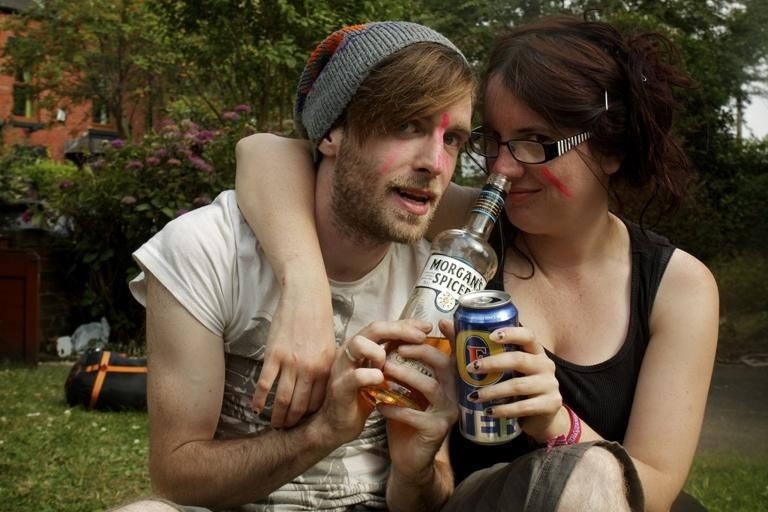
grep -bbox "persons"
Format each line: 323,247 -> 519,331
232,10 -> 721,512
107,19 -> 645,511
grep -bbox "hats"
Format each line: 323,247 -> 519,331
290,17 -> 475,160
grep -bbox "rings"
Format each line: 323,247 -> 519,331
342,344 -> 359,364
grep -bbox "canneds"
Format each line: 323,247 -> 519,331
452,289 -> 522,446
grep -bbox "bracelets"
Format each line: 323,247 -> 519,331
525,400 -> 581,455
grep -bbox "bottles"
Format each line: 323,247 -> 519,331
361,170 -> 512,411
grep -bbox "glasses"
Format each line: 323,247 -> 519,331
464,121 -> 594,164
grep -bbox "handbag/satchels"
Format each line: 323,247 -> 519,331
64,347 -> 148,413
